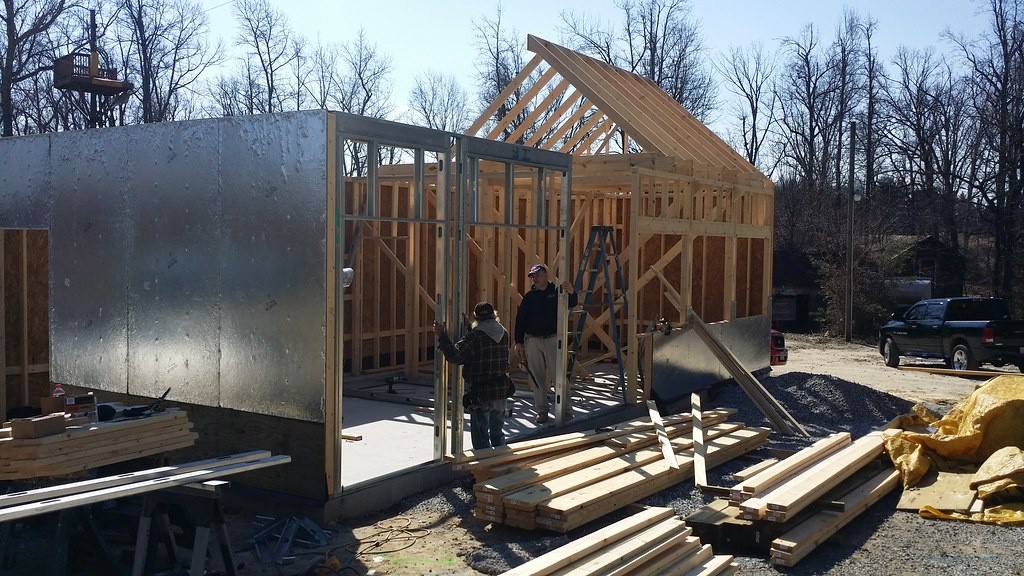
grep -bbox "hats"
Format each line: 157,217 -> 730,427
528,265 -> 547,277
473,301 -> 494,319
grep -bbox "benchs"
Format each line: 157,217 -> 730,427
49,465 -> 235,576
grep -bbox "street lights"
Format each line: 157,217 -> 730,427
845,189 -> 861,342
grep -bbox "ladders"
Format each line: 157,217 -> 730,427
567,225 -> 644,405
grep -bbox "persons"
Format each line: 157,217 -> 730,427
514,264 -> 578,423
433,302 -> 516,449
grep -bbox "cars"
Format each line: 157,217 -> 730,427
770,329 -> 788,366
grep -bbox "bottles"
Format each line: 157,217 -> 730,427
386,376 -> 407,383
52,384 -> 65,398
85,391 -> 98,429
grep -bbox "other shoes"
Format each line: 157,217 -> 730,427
565,409 -> 573,419
535,412 -> 548,423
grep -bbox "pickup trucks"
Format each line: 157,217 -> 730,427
877,297 -> 1024,376
